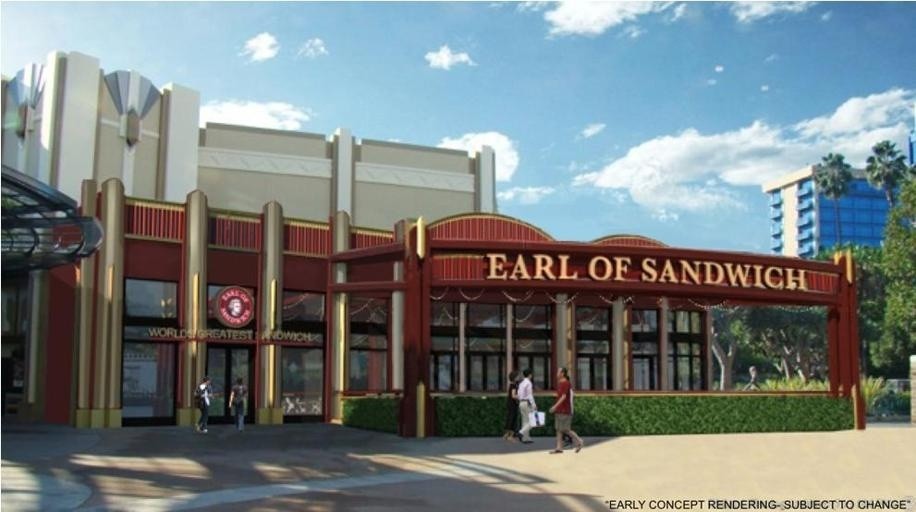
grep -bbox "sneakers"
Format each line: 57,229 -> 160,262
195,423 -> 208,434
503,432 -> 534,444
549,449 -> 563,453
576,439 -> 584,452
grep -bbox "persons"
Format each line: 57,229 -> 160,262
549,365 -> 586,454
228,376 -> 250,428
560,376 -> 575,448
745,366 -> 763,391
192,376 -> 215,434
502,371 -> 519,442
515,368 -> 539,443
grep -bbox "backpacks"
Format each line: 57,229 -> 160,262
194,395 -> 204,409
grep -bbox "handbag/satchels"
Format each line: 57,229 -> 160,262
529,411 -> 546,427
233,395 -> 242,406
